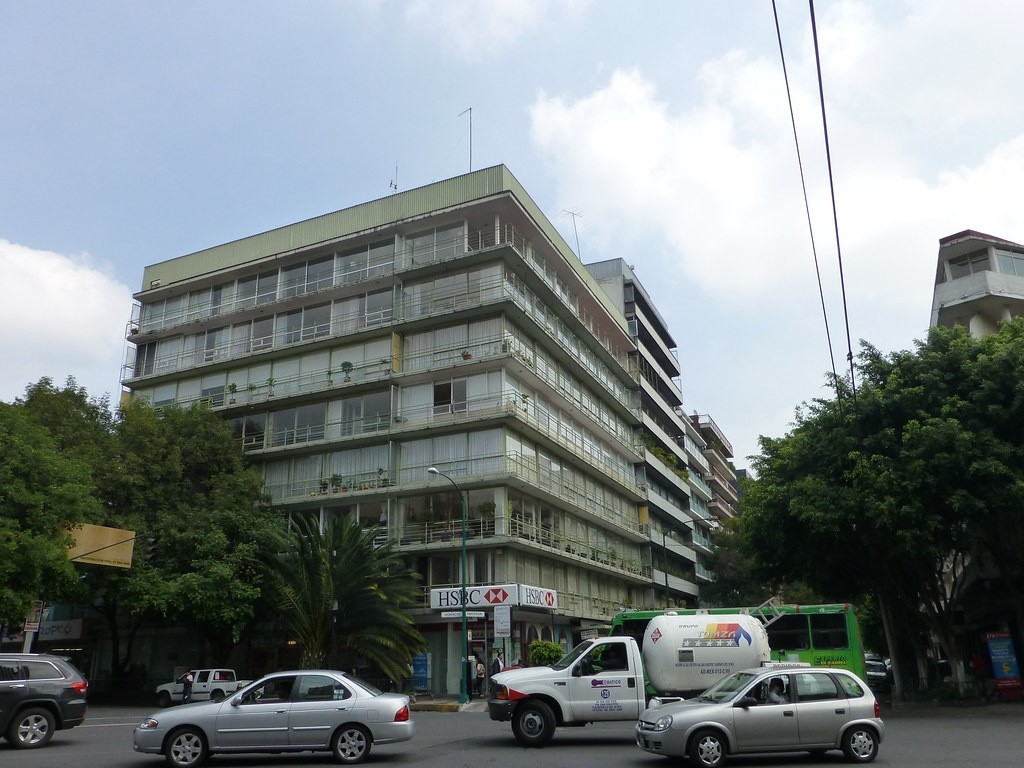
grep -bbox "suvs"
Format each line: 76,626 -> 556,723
0,652 -> 88,748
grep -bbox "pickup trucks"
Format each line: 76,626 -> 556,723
155,669 -> 264,708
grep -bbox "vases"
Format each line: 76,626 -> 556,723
578,553 -> 587,559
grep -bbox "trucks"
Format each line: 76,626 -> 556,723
488,611 -> 773,746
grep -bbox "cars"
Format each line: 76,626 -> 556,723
865,661 -> 887,690
634,663 -> 886,768
133,669 -> 415,768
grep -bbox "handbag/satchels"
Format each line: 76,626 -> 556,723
477,664 -> 485,675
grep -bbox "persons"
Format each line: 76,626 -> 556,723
492,653 -> 504,675
766,678 -> 788,704
475,659 -> 485,698
588,646 -> 623,669
182,670 -> 194,704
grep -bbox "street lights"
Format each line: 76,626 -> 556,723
662,515 -> 719,609
429,469 -> 469,703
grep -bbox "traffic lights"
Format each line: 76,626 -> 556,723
144,537 -> 157,562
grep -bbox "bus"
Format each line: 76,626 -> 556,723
592,602 -> 870,700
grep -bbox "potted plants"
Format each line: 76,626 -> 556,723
228,382 -> 237,404
266,378 -> 276,397
329,473 -> 348,493
502,338 -> 512,353
601,546 -> 617,567
320,480 -> 328,495
341,361 -> 354,383
619,556 -> 639,574
460,349 -> 472,360
590,544 -> 601,562
566,545 -> 576,553
